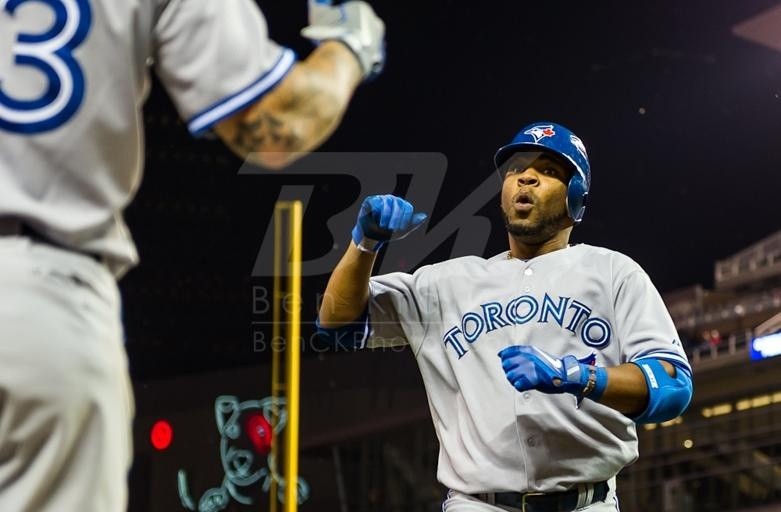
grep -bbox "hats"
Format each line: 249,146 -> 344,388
494,119 -> 594,225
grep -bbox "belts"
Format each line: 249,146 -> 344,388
1,210 -> 103,266
472,481 -> 609,512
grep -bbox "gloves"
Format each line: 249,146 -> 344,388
498,342 -> 608,399
303,1 -> 388,78
349,191 -> 428,253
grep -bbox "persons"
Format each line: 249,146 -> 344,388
2,0 -> 386,512
317,120 -> 694,510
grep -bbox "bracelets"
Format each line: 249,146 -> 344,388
574,365 -> 597,401
